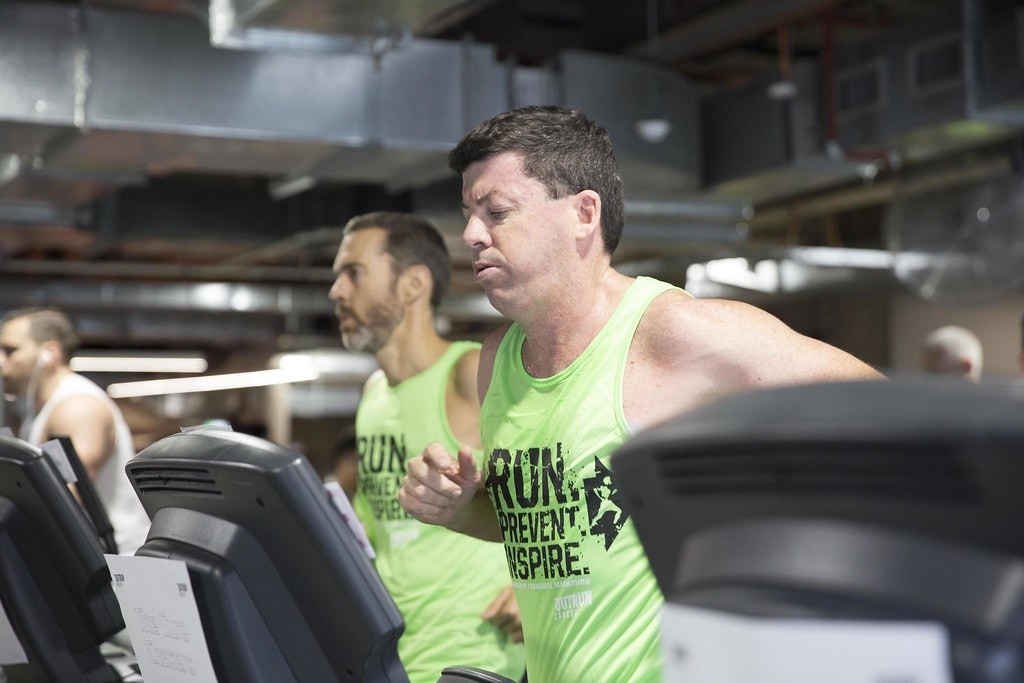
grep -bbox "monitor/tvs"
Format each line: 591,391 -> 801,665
0,371 -> 1024,683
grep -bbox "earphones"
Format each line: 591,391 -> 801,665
38,349 -> 51,366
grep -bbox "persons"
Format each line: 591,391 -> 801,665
396,103 -> 894,683
916,324 -> 986,388
318,213 -> 529,683
325,422 -> 359,508
0,298 -> 153,557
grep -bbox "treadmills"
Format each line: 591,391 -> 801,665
102,420 -> 428,683
613,373 -> 1023,683
0,424 -> 152,683
